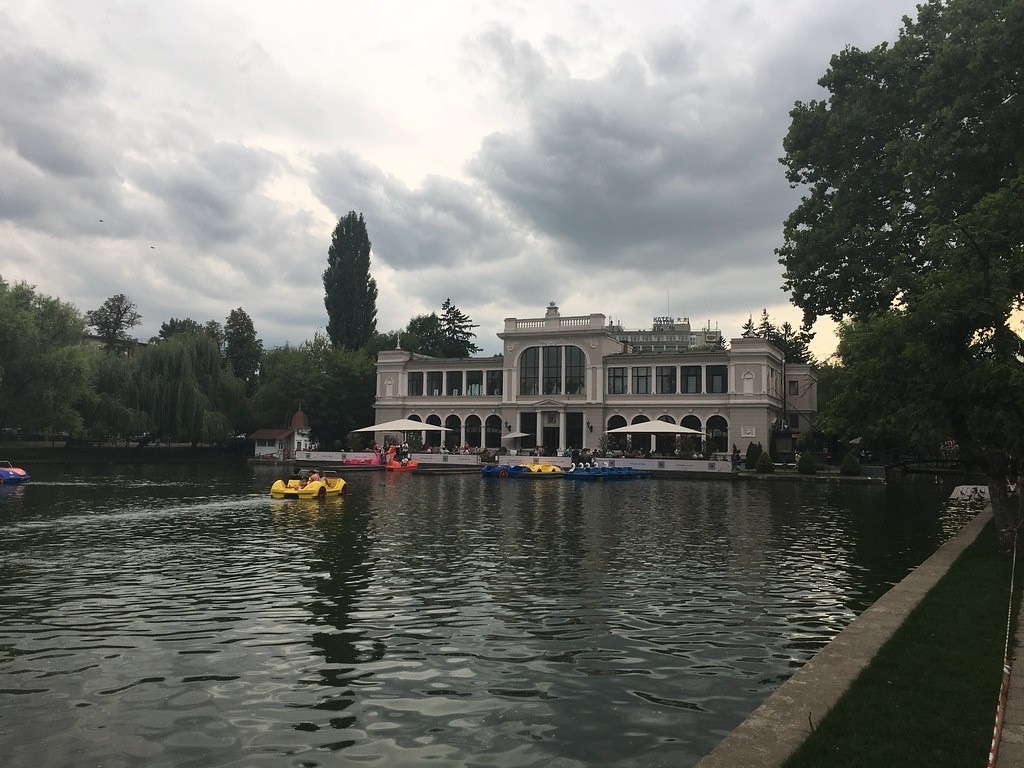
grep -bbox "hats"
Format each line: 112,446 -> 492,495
403,441 -> 408,444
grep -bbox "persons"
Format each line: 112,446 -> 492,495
731,448 -> 742,472
586,448 -> 592,456
463,448 -> 470,455
465,442 -> 469,450
309,469 -> 319,482
396,442 -> 401,457
793,450 -> 802,470
401,455 -> 408,466
288,468 -> 303,480
402,441 -> 409,457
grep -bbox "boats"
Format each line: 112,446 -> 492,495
0,461 -> 32,484
482,462 -> 651,478
343,444 -> 420,469
271,470 -> 348,499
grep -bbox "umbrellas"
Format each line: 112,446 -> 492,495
602,420 -> 708,435
500,431 -> 530,450
849,437 -> 862,444
352,418 -> 453,443
